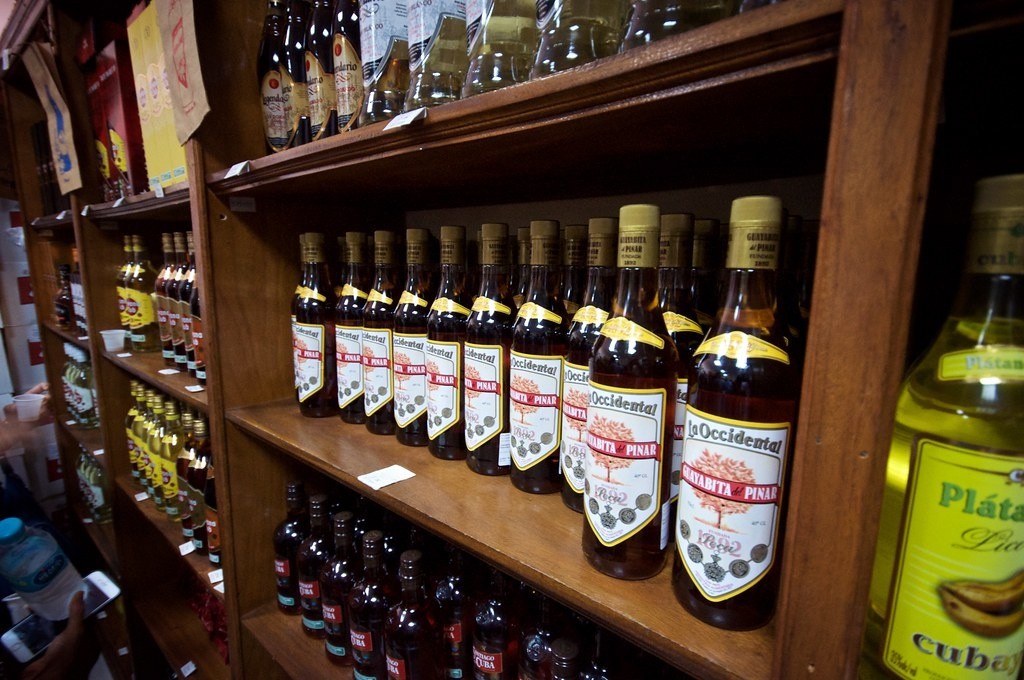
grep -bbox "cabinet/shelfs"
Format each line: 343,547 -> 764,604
0,1 -> 1024,680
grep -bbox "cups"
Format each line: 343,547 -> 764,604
11,394 -> 45,422
99,330 -> 126,353
2,593 -> 30,626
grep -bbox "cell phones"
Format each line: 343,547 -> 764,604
0,571 -> 123,665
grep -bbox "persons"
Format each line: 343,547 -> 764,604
0,382 -> 56,453
20,592 -> 84,680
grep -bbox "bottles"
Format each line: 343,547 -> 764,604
256,0 -> 799,631
272,483 -> 624,680
54,229 -> 222,568
852,169 -> 1023,680
0,517 -> 88,620
